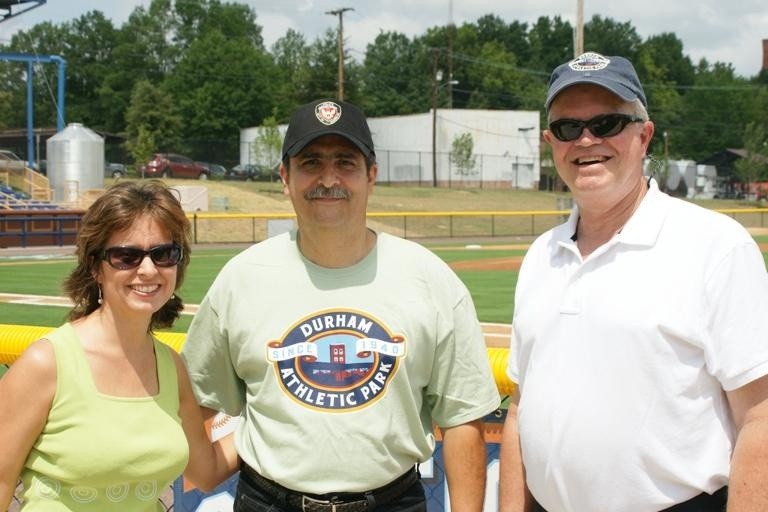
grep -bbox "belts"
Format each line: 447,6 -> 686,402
236,460 -> 422,512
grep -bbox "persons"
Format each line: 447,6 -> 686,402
0,179 -> 243,512
496,50 -> 768,512
176,99 -> 502,511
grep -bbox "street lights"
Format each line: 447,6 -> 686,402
432,70 -> 459,187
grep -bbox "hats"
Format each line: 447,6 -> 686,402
540,51 -> 649,112
280,96 -> 374,161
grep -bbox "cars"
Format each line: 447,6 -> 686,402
1,151 -> 29,170
136,153 -> 281,180
104,159 -> 123,178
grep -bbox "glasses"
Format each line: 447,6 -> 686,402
88,243 -> 189,272
545,113 -> 644,144
